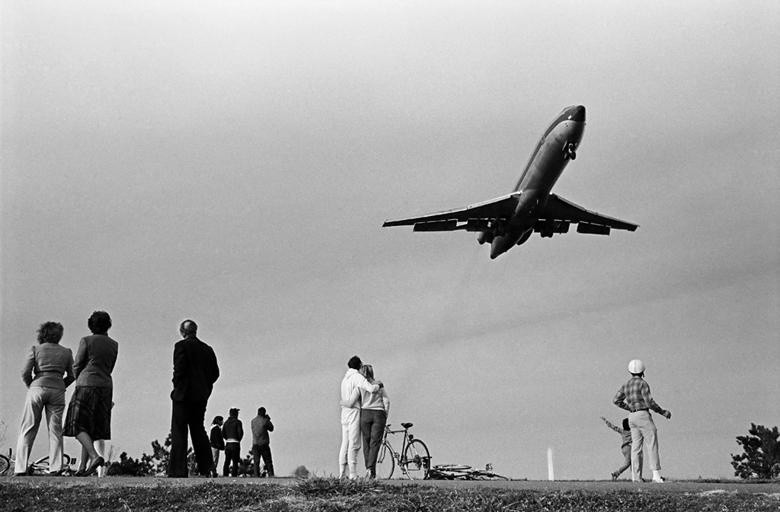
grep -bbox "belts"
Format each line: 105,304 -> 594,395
630,409 -> 646,412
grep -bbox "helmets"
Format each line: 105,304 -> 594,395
628,359 -> 644,374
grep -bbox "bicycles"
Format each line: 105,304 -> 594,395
374,422 -> 433,480
0,448 -> 75,478
428,463 -> 510,483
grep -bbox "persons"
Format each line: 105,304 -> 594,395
11,319 -> 76,476
207,414 -> 224,475
338,362 -> 391,482
599,414 -> 632,481
336,354 -> 385,481
250,406 -> 276,478
611,358 -> 673,485
162,318 -> 221,479
62,309 -> 121,476
222,407 -> 245,477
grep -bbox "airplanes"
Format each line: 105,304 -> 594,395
382,105 -> 640,261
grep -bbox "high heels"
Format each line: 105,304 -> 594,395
83,457 -> 104,476
73,468 -> 85,476
